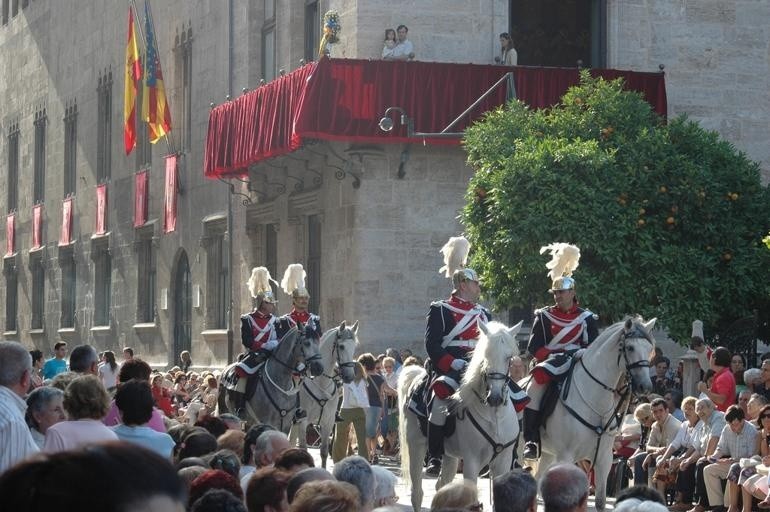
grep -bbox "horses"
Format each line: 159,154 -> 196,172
393,316 -> 525,512
288,320 -> 362,468
511,314 -> 659,512
218,316 -> 324,437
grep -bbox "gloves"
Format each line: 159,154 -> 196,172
450,359 -> 466,371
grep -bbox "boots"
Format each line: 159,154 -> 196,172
334,396 -> 345,422
235,391 -> 246,418
523,407 -> 542,459
295,391 -> 307,419
425,421 -> 445,475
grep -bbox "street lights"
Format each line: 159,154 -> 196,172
379,71 -> 517,140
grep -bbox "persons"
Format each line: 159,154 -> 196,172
747,393 -> 768,425
167,423 -> 188,443
164,373 -> 172,382
205,373 -> 214,378
193,417 -> 228,440
27,387 -> 69,448
493,469 -> 538,512
702,405 -> 759,512
627,403 -> 657,487
52,371 -> 80,392
371,465 -> 409,512
633,398 -> 683,503
431,481 -> 479,512
612,499 -> 669,512
656,397 -> 701,512
420,269 -> 525,476
153,375 -> 176,418
614,486 -> 666,512
290,481 -> 362,512
42,342 -> 67,380
333,455 -> 377,512
690,336 -> 713,361
189,470 -> 243,506
44,374 -> 119,458
289,468 -> 337,503
647,393 -> 662,402
663,390 -> 688,422
541,464 -> 589,512
679,399 -> 726,512
181,426 -> 211,440
201,371 -> 210,377
276,288 -> 323,342
96,352 -> 105,368
376,354 -> 387,372
332,360 -> 368,463
699,370 -> 718,400
744,369 -> 764,392
168,370 -> 175,378
177,457 -> 206,470
99,350 -> 121,389
650,357 -> 673,395
588,399 -> 641,496
273,448 -> 315,475
120,358 -> 151,382
650,346 -> 670,380
756,359 -> 770,402
381,29 -> 400,61
183,375 -> 213,408
189,488 -> 248,511
218,413 -> 244,430
381,357 -> 399,453
184,378 -> 219,424
523,275 -> 600,460
738,389 -> 753,421
181,434 -> 219,459
218,430 -> 248,455
238,424 -> 279,477
734,370 -> 747,400
402,350 -> 412,361
180,350 -> 193,374
387,348 -> 403,366
123,348 -> 134,361
0,340 -> 41,473
696,347 -> 736,412
175,371 -> 183,378
186,374 -> 199,394
757,458 -> 770,509
176,465 -> 210,501
193,376 -> 204,395
186,371 -> 193,380
228,290 -> 282,415
0,440 -> 185,512
726,404 -> 770,512
358,353 -> 397,465
27,348 -> 42,394
673,359 -> 684,395
210,449 -> 241,478
372,505 -> 407,512
108,379 -> 178,463
730,354 -> 746,373
382,25 -> 413,61
172,366 -> 182,374
247,468 -> 289,512
404,355 -> 419,367
174,374 -> 190,407
500,33 -> 517,66
240,431 -> 289,509
510,356 -> 523,381
71,344 -> 99,375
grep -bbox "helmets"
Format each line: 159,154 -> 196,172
282,264 -> 310,304
540,242 -> 581,297
439,237 -> 480,294
249,267 -> 277,310
